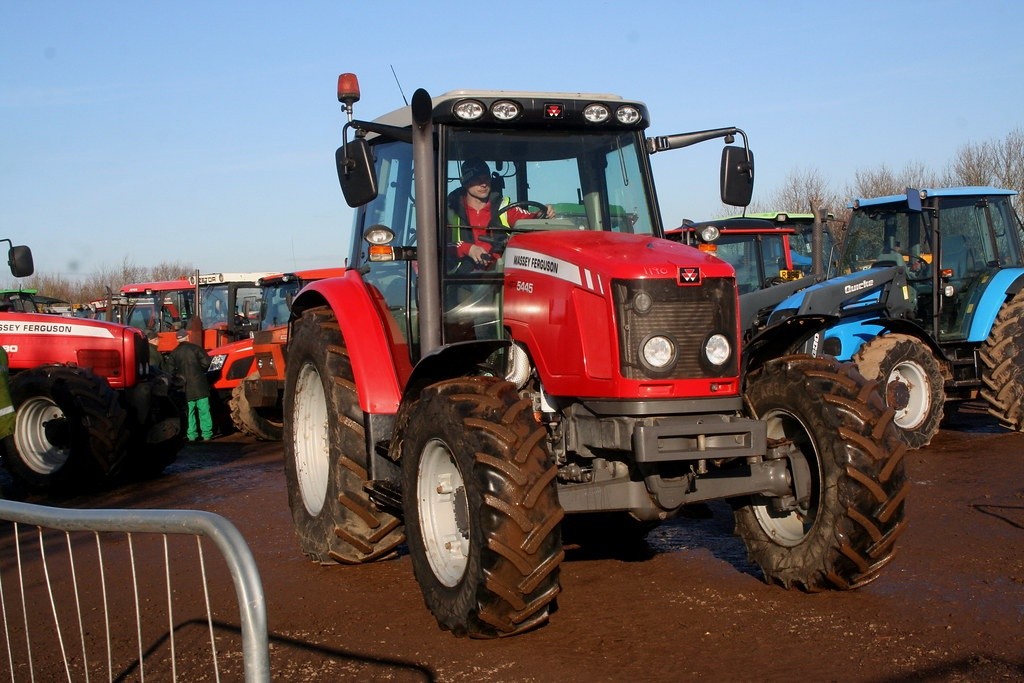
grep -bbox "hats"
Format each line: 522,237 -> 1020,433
175,328 -> 189,341
146,332 -> 164,340
459,155 -> 490,185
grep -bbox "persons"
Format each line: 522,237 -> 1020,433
146,331 -> 166,374
166,329 -> 212,440
443,157 -> 555,340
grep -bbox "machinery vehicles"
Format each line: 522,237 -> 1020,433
0,240 -> 347,500
653,210 -> 844,309
279,70 -> 899,639
747,185 -> 1024,456
